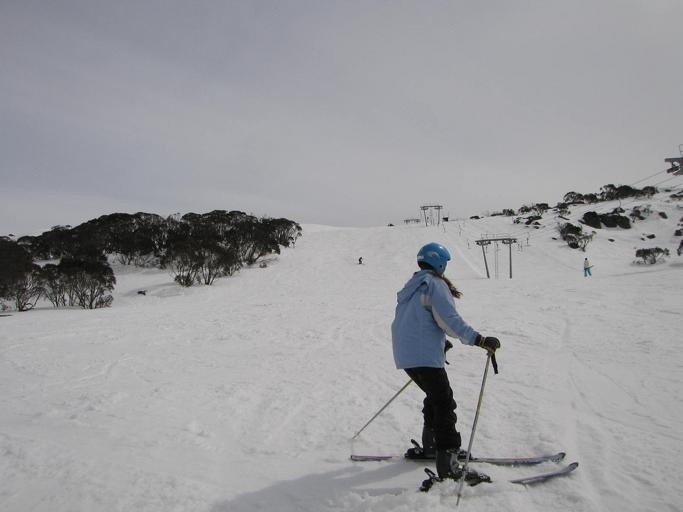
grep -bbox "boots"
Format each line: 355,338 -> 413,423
436,448 -> 478,479
423,425 -> 471,454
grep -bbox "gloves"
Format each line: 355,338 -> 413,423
475,334 -> 500,353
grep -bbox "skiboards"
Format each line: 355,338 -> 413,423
350,453 -> 578,494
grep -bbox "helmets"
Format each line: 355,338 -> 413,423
417,243 -> 451,275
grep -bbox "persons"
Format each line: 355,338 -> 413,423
583,256 -> 592,277
358,256 -> 362,263
389,242 -> 500,486
